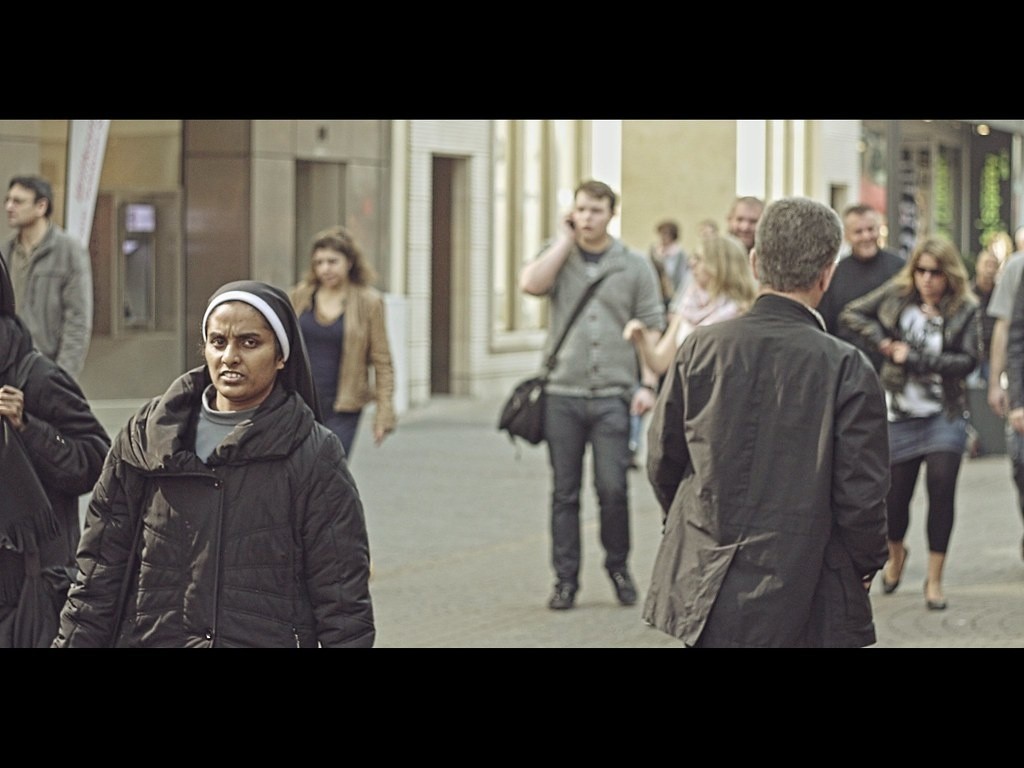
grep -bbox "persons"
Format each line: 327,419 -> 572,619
0,174 -> 93,382
285,227 -> 397,461
519,182 -> 667,611
51,281 -> 377,648
621,196 -> 1024,612
646,198 -> 892,648
0,252 -> 113,648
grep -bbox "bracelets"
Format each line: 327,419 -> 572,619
640,383 -> 658,393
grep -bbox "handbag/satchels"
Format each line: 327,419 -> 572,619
498,377 -> 547,443
878,356 -> 908,391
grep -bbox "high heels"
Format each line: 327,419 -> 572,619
922,580 -> 947,610
882,548 -> 907,593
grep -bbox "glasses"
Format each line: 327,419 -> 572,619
913,265 -> 945,276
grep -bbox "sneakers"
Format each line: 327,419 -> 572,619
610,568 -> 638,604
549,580 -> 579,609
